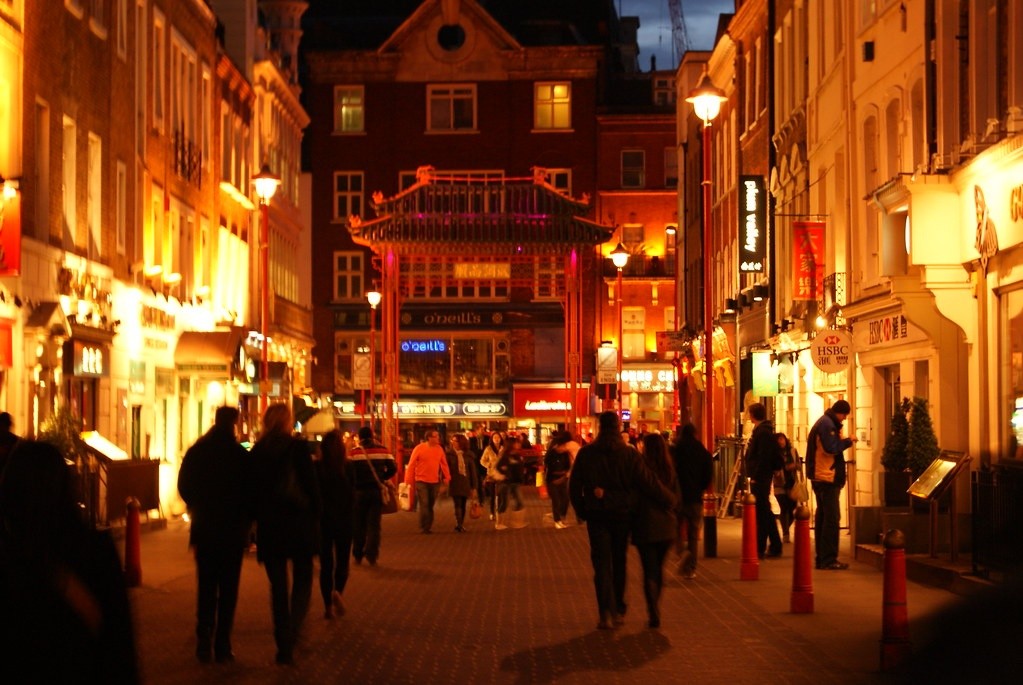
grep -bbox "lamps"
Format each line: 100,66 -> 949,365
781,319 -> 795,332
745,290 -> 754,304
737,294 -> 753,311
724,298 -> 742,315
771,324 -> 782,336
753,285 -> 768,301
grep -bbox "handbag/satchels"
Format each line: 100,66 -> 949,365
399,483 -> 414,511
470,498 -> 481,518
381,479 -> 399,514
789,480 -> 809,502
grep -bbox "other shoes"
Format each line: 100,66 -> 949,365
325,590 -> 346,623
816,560 -> 849,569
597,609 -> 623,628
425,529 -> 432,534
555,522 -> 567,529
649,609 -> 659,628
783,535 -> 790,544
674,551 -> 696,579
490,514 -> 495,520
455,524 -> 465,532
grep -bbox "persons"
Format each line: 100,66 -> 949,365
0,442 -> 140,685
745,403 -> 785,558
406,429 -> 450,533
468,438 -> 486,508
178,405 -> 250,665
0,412 -> 26,467
251,403 -> 316,667
447,435 -> 478,532
587,424 -> 674,455
568,411 -> 643,631
480,429 -> 538,521
806,400 -> 858,569
317,431 -> 356,617
346,426 -> 398,564
544,430 -> 586,528
673,424 -> 714,579
595,434 -> 681,626
773,434 -> 800,543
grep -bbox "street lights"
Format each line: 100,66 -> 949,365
611,243 -> 631,429
686,72 -> 728,558
251,161 -> 283,435
367,289 -> 383,433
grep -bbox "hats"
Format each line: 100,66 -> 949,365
832,399 -> 851,414
